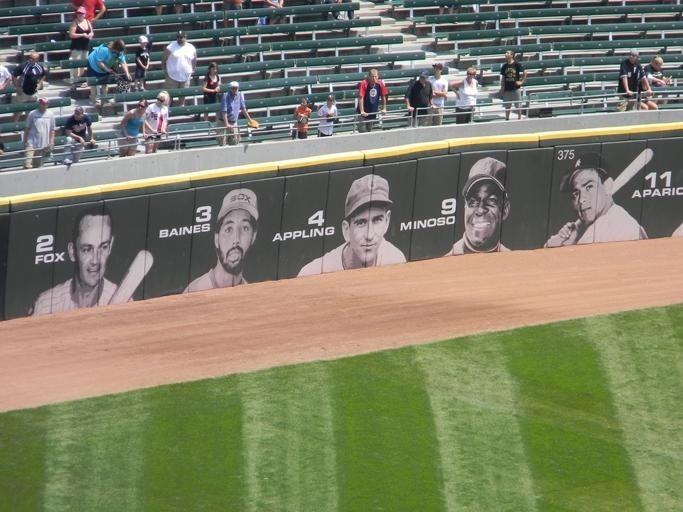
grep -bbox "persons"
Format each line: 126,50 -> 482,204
328,0 -> 360,20
543,152 -> 648,249
24,205 -> 133,316
296,174 -> 406,278
617,49 -> 670,112
267,2 -> 285,25
293,50 -> 527,138
180,188 -> 259,294
1,29 -> 254,167
69,1 -> 106,87
442,157 -> 510,257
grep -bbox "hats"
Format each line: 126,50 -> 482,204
432,63 -> 442,69
420,71 -> 429,78
560,152 -> 607,192
462,157 -> 508,197
345,174 -> 393,217
37,8 -> 239,104
216,187 -> 259,224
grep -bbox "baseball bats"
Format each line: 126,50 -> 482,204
108,250 -> 152,305
549,148 -> 653,245
